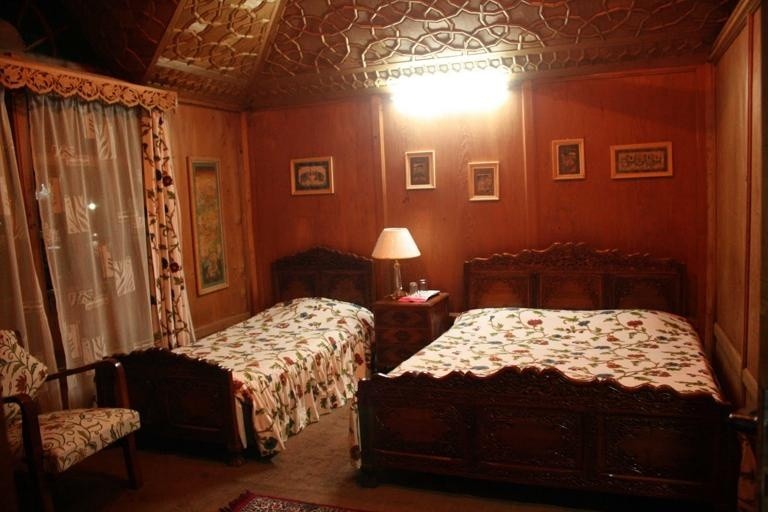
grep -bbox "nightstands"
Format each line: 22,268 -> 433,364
369,289 -> 450,372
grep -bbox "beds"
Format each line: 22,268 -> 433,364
93,244 -> 372,467
356,241 -> 726,512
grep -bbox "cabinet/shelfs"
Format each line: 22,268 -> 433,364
704,0 -> 751,423
740,0 -> 768,428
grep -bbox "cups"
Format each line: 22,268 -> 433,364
409,282 -> 419,299
419,278 -> 429,295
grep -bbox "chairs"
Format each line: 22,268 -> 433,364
1,330 -> 142,512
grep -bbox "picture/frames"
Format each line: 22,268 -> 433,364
551,138 -> 586,180
190,160 -> 230,297
467,161 -> 500,203
609,142 -> 673,178
404,150 -> 437,190
289,156 -> 336,195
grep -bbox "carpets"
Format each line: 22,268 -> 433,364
218,491 -> 361,512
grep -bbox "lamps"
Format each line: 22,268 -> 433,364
372,227 -> 421,300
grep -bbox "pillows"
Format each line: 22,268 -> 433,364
1,330 -> 48,426
